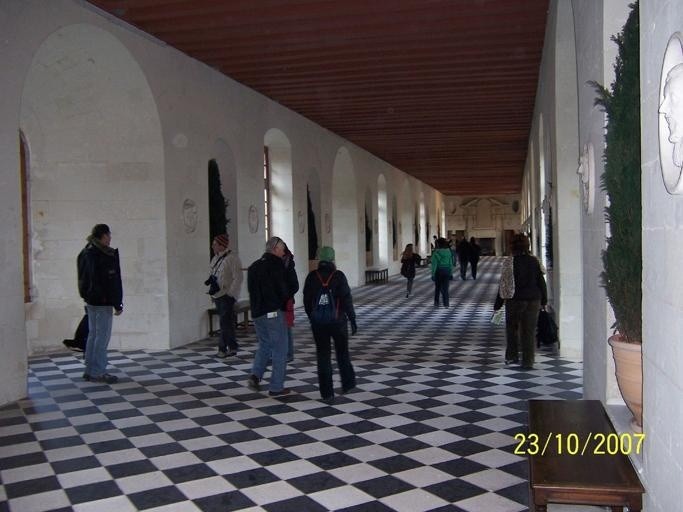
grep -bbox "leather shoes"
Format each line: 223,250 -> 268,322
268,386 -> 290,396
247,374 -> 260,391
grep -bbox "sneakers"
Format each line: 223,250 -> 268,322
341,383 -> 356,393
265,357 -> 294,367
215,347 -> 237,358
322,392 -> 334,403
83,372 -> 119,382
405,262 -> 476,309
521,362 -> 533,369
505,358 -> 518,364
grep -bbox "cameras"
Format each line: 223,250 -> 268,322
205,276 -> 217,285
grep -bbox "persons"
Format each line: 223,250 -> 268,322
247,236 -> 290,396
303,246 -> 357,404
493,233 -> 547,370
430,239 -> 453,309
208,234 -> 243,358
401,243 -> 417,298
432,235 -> 480,281
77,223 -> 123,383
282,242 -> 299,364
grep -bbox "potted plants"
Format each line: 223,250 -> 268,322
305,181 -> 320,271
583,0 -> 648,428
364,203 -> 371,262
543,182 -> 554,291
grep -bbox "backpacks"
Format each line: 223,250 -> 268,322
536,305 -> 558,348
313,269 -> 340,325
62,305 -> 88,359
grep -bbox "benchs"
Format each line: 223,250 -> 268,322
522,398 -> 648,512
209,299 -> 256,339
365,266 -> 388,283
419,258 -> 428,268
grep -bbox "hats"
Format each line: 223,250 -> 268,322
213,233 -> 229,248
